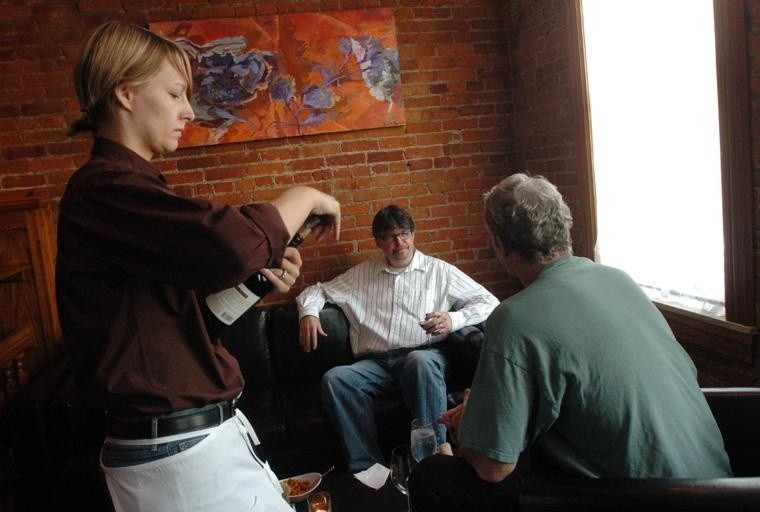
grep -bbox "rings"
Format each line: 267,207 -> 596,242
279,269 -> 288,281
439,322 -> 443,329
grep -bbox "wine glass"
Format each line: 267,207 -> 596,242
410,418 -> 441,462
391,446 -> 416,511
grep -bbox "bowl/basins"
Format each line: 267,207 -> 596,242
279,471 -> 322,503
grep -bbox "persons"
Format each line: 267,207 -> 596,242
406,172 -> 737,504
295,203 -> 503,474
53,19 -> 343,512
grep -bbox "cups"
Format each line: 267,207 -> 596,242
306,491 -> 332,512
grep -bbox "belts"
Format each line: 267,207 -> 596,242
105,401 -> 236,440
354,342 -> 441,360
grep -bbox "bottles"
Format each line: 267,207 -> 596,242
197,216 -> 321,343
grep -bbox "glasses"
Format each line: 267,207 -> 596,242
378,229 -> 412,244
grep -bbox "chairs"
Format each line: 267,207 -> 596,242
514,380 -> 759,508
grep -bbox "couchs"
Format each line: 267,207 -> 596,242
15,284 -> 496,488
